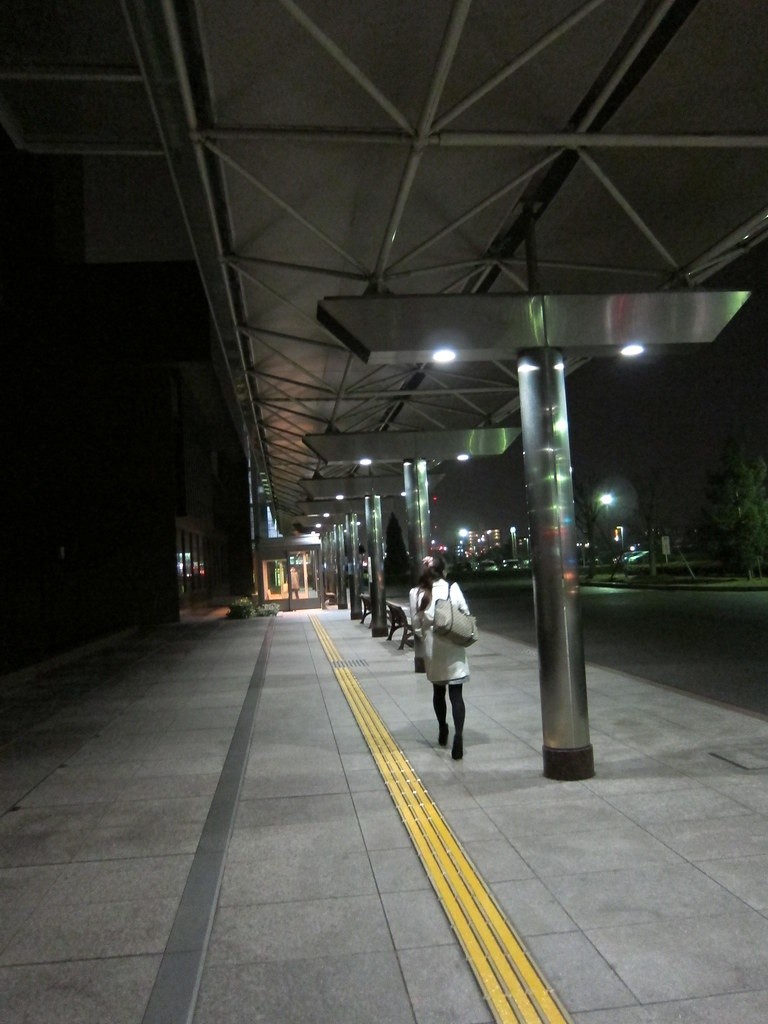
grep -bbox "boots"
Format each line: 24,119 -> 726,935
438,724 -> 449,746
452,735 -> 464,759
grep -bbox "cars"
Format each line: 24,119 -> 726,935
478,561 -> 499,573
613,550 -> 651,564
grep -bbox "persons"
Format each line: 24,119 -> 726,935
410,553 -> 471,760
290,565 -> 299,599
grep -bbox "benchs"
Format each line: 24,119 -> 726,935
384,599 -> 416,650
361,595 -> 374,628
324,591 -> 336,605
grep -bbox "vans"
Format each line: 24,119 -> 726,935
503,559 -> 521,570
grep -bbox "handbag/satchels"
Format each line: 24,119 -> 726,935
434,581 -> 477,648
